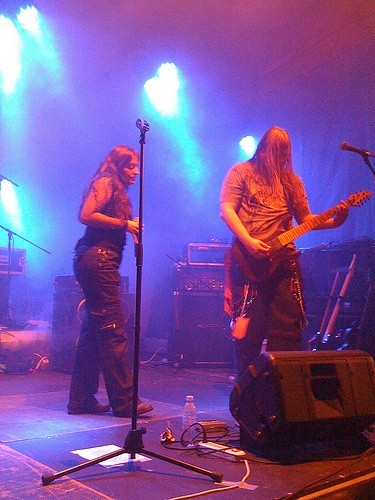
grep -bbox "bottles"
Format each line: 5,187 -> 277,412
182,395 -> 198,447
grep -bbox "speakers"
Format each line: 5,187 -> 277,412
170,289 -> 242,369
228,349 -> 374,455
46,291 -> 136,376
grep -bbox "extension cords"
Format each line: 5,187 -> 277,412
198,442 -> 246,463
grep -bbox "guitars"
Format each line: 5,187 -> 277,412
232,189 -> 374,284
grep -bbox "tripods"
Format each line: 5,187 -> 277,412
41,121 -> 223,484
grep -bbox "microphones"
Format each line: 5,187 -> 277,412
339,142 -> 375,158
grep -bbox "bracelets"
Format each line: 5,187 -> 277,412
120,219 -> 128,230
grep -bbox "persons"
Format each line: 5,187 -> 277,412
68,145 -> 154,415
219,126 -> 350,377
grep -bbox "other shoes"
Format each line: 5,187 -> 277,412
68,397 -> 109,414
113,402 -> 153,417
